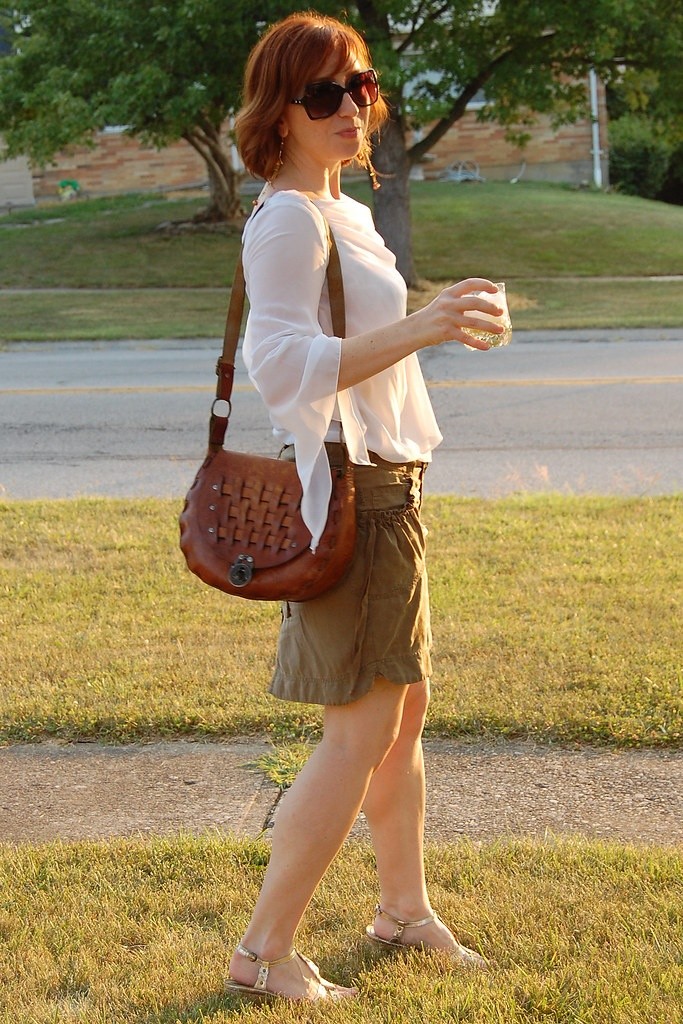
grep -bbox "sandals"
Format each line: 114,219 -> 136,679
364,905 -> 492,973
224,945 -> 339,1006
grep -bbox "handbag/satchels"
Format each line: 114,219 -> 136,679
178,444 -> 358,601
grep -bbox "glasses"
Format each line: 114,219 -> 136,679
286,68 -> 381,120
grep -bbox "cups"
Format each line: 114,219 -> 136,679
461,283 -> 512,351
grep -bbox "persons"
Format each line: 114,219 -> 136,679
224,12 -> 502,1000
